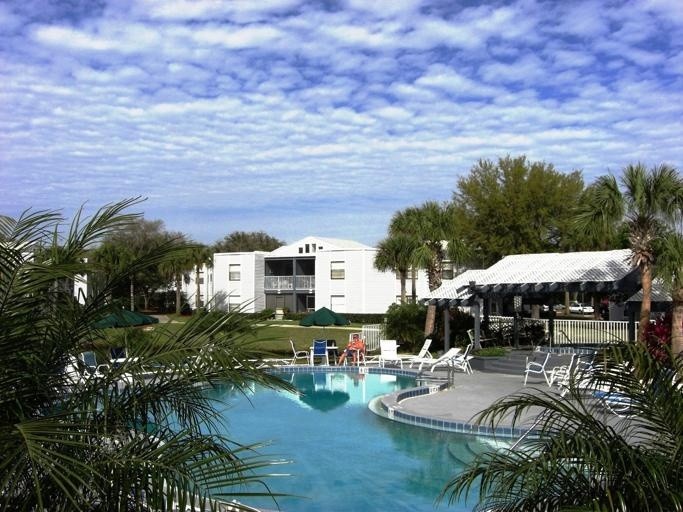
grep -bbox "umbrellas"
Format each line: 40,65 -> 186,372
93,309 -> 158,357
300,306 -> 351,339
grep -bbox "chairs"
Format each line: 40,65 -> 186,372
522,351 -> 677,420
63,352 -> 133,396
287,335 -> 474,376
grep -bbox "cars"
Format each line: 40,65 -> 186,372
568,300 -> 595,314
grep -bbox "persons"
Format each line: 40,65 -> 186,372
337,334 -> 364,366
